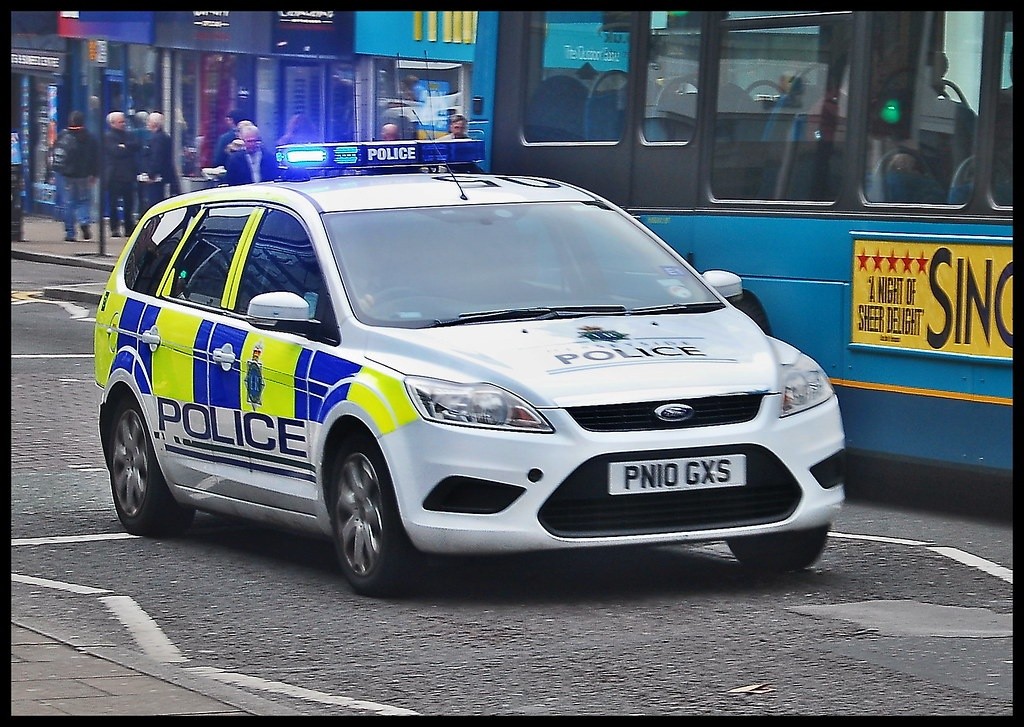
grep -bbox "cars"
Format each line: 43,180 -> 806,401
94,140 -> 845,596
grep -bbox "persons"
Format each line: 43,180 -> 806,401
50,109 -> 99,241
365,123 -> 420,173
103,113 -> 138,236
229,126 -> 279,189
428,116 -> 476,174
221,119 -> 254,175
127,110 -> 150,202
212,107 -> 247,173
136,113 -> 171,218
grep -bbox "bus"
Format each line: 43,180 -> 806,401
466,0 -> 1020,491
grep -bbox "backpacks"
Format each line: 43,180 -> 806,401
48,129 -> 79,175
